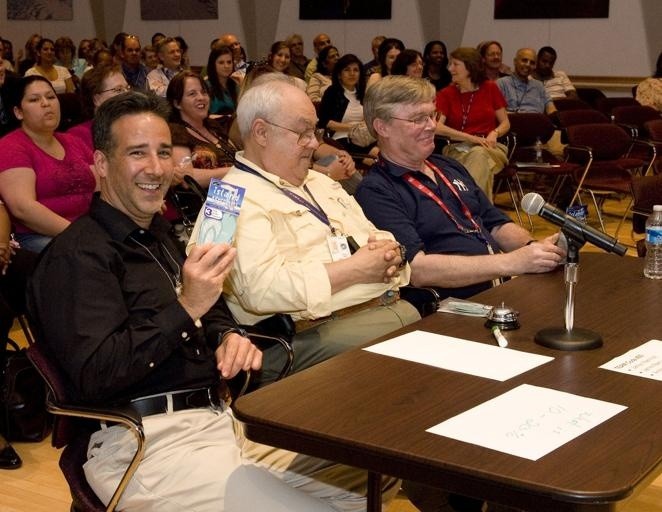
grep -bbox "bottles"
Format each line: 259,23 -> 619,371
643,204 -> 662,281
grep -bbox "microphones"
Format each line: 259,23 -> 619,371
522,192 -> 628,257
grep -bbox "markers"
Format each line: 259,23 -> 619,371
491,325 -> 508,348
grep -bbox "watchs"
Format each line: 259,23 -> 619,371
398,244 -> 408,268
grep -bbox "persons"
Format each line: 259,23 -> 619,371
355,74 -> 568,320
1,32 -> 576,221
0,75 -> 102,254
24,91 -> 370,512
636,51 -> 662,111
184,78 -> 423,503
0,200 -> 23,470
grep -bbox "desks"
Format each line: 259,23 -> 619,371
231,251 -> 662,512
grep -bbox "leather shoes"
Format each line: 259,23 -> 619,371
0,445 -> 21,469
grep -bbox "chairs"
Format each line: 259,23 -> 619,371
25,324 -> 296,512
491,86 -> 661,249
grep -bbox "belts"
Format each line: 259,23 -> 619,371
295,290 -> 400,333
92,388 -> 220,433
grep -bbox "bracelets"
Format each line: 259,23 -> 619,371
220,327 -> 247,343
526,240 -> 538,245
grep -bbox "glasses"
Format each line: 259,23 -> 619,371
390,112 -> 440,129
264,121 -> 321,147
101,85 -> 130,94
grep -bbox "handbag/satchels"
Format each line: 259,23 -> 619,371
166,175 -> 209,247
349,121 -> 377,147
0,339 -> 55,443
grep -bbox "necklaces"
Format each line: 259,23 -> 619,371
130,236 -> 202,328
